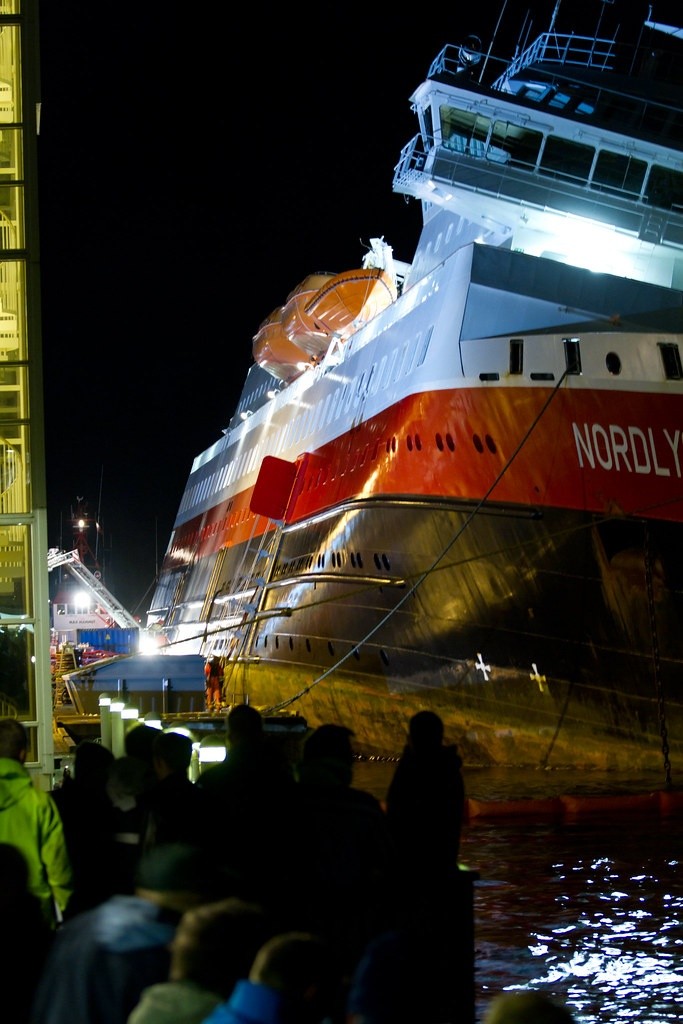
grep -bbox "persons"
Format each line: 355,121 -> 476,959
0,703 -> 468,1024
205,656 -> 223,712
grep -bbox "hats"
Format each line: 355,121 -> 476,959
134,840 -> 226,893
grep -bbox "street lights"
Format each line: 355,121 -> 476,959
78,519 -> 85,564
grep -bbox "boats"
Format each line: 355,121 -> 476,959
141,0 -> 682,823
